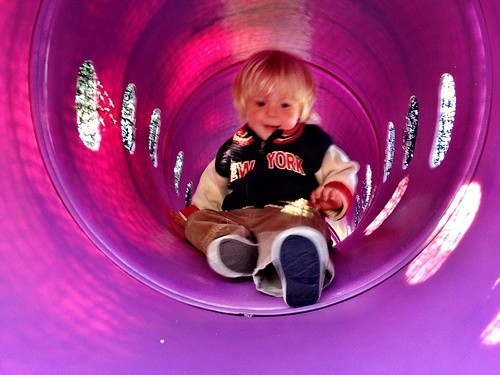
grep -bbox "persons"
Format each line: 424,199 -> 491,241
169,49 -> 360,307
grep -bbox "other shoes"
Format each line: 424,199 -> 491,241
271,226 -> 329,307
206,234 -> 259,278
